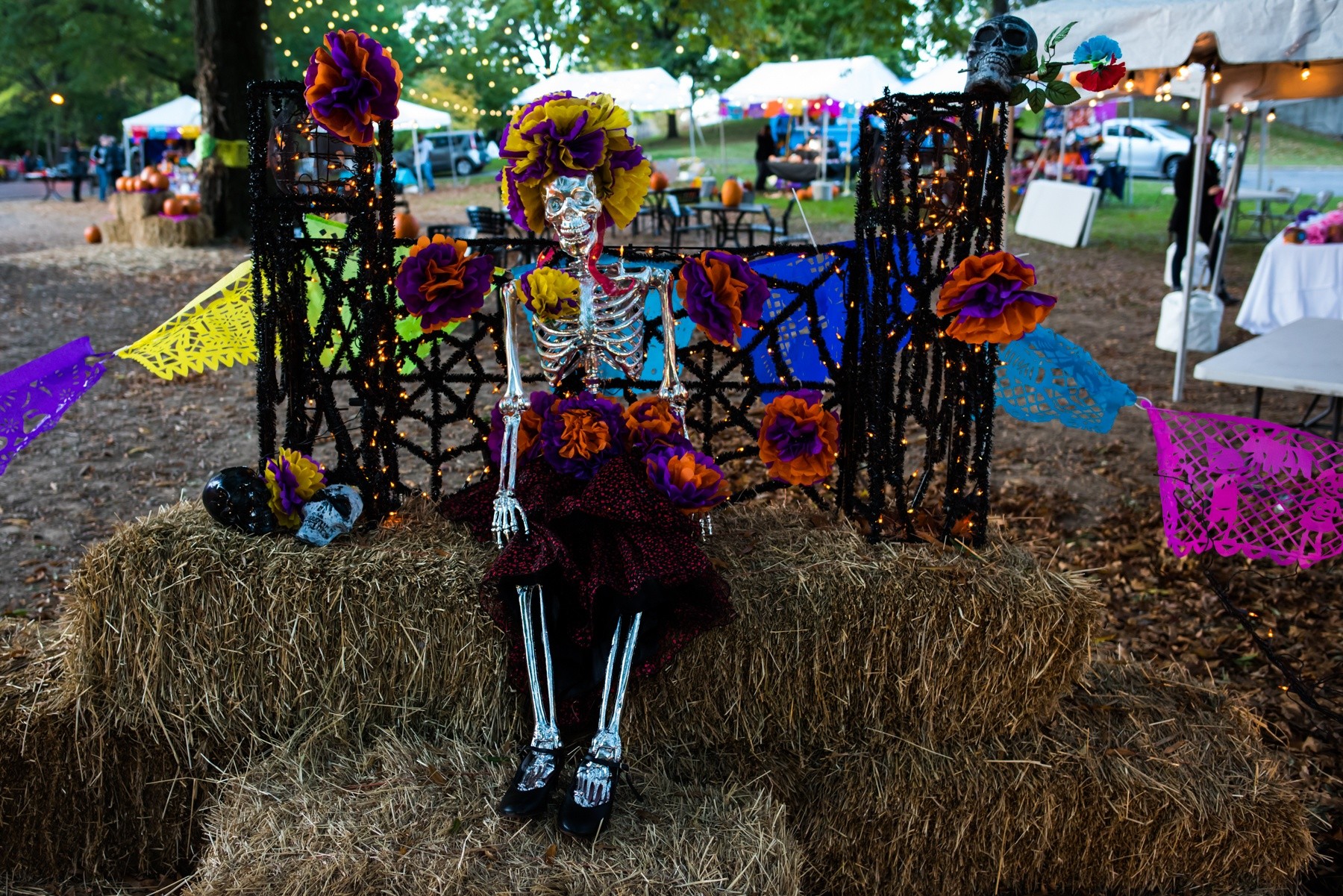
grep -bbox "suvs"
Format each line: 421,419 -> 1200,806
770,107 -> 951,167
393,129 -> 489,174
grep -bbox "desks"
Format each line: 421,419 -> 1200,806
766,156 -> 859,186
1194,317 -> 1343,447
1237,214 -> 1343,338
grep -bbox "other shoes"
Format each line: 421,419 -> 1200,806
1215,294 -> 1240,306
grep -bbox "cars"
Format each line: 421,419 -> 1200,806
1090,116 -> 1240,176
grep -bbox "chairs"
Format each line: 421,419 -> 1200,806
1233,182 -> 1334,250
423,204 -> 533,246
630,183 -> 794,248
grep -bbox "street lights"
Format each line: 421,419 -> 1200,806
51,91 -> 83,205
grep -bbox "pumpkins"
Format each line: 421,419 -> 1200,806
721,175 -> 743,206
795,181 -> 839,203
83,226 -> 100,242
115,166 -> 169,192
393,209 -> 420,241
162,199 -> 195,215
650,169 -> 668,193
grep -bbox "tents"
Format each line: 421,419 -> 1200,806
894,58 -> 1074,110
302,95 -> 456,198
997,0 -> 1343,413
497,61 -> 701,160
119,95 -> 204,184
719,51 -> 902,201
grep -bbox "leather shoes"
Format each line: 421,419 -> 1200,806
558,753 -> 644,836
499,734 -> 566,816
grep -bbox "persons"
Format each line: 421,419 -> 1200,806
14,131 -> 127,206
1009,115 -> 1048,163
408,129 -> 435,192
1168,127 -> 1244,306
751,123 -> 785,195
